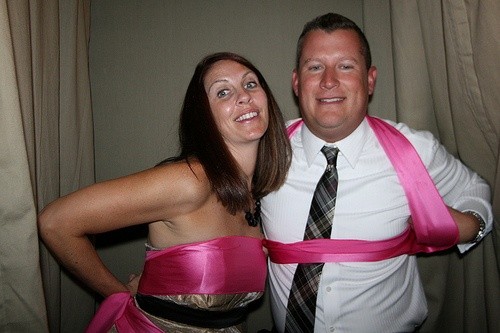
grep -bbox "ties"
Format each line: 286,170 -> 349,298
283,146 -> 339,333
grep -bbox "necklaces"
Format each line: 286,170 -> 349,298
243,197 -> 263,227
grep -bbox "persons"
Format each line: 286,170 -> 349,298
36,51 -> 294,332
257,11 -> 494,332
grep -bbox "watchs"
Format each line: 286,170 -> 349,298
466,210 -> 485,244
